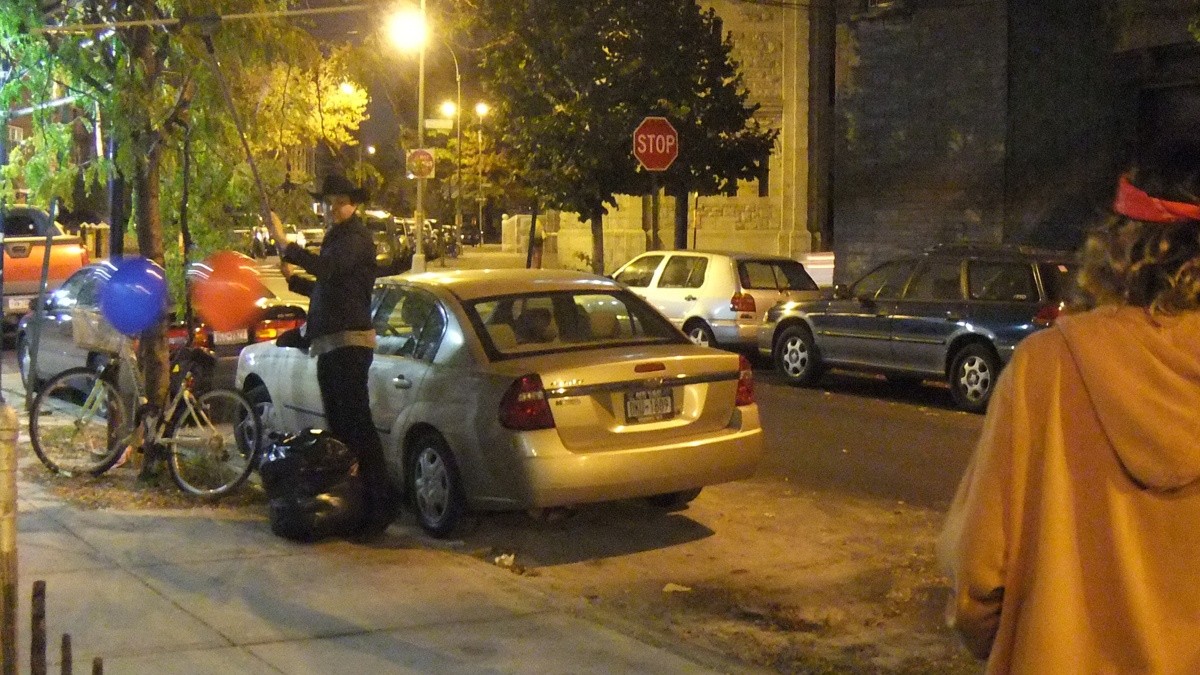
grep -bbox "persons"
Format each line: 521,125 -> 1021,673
269,175 -> 385,473
531,217 -> 547,268
933,154 -> 1200,675
470,216 -> 480,248
252,222 -> 270,258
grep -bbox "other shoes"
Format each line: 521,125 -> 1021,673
360,502 -> 401,540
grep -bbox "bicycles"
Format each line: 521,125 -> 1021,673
445,228 -> 463,257
29,333 -> 263,500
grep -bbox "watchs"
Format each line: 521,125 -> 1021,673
278,251 -> 286,259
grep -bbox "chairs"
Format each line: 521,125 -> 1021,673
587,312 -> 623,339
978,269 -> 1018,299
513,306 -> 555,342
401,297 -> 436,346
1031,274 -> 1063,302
662,264 -> 694,288
487,324 -> 516,356
929,275 -> 962,301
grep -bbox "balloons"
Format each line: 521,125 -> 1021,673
194,250 -> 264,333
103,256 -> 168,336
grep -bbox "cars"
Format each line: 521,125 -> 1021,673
234,268 -> 763,540
13,255 -> 310,428
225,207 -> 479,277
757,241 -> 1090,413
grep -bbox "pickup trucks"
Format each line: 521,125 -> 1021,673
2,203 -> 90,349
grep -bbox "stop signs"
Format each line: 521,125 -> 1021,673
632,117 -> 678,171
405,147 -> 437,180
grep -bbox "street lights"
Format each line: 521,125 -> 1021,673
476,101 -> 486,245
390,11 -> 463,228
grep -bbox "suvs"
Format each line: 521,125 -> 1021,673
608,250 -> 823,351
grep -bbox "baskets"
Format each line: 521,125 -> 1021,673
69,303 -> 119,354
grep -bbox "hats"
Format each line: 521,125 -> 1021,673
309,175 -> 369,203
